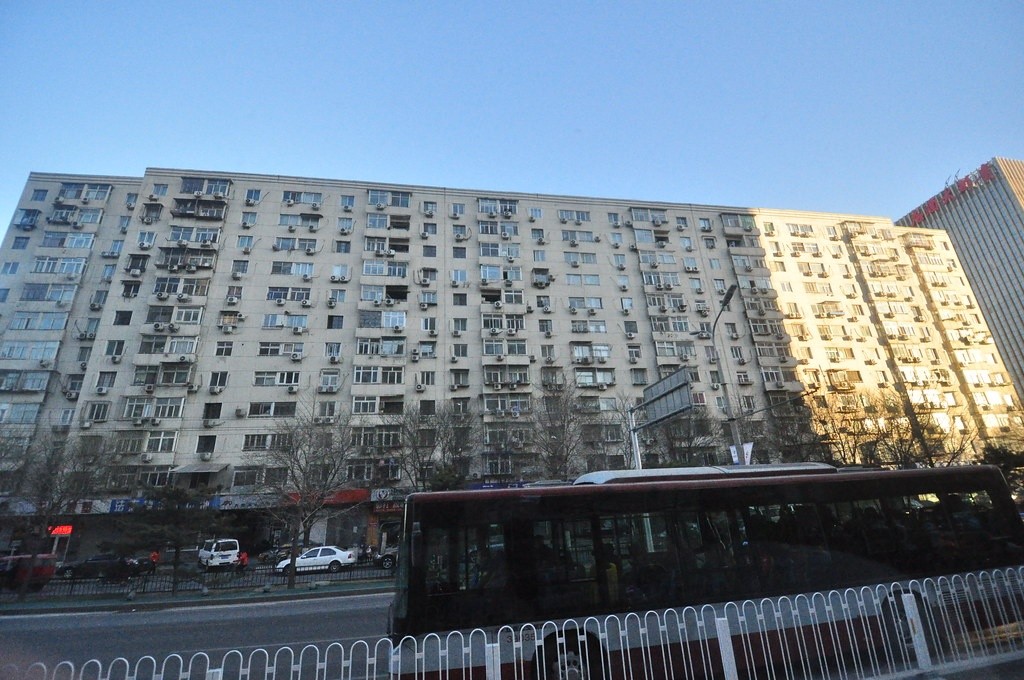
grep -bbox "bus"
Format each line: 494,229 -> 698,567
387,469 -> 1021,680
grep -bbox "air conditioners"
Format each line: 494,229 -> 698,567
373,277 -> 432,311
453,276 -> 513,308
341,201 -> 395,256
568,216 -> 1016,446
276,272 -> 351,309
293,326 -> 303,336
412,347 -> 459,423
545,331 -> 553,338
394,324 -> 404,333
421,207 -> 464,240
494,355 -> 565,449
274,197 -> 322,256
0,192 -> 256,466
429,329 -> 439,337
453,330 -> 462,337
488,208 -> 545,262
526,274 -> 557,313
289,352 -> 340,458
493,328 -> 517,337
560,217 -> 567,223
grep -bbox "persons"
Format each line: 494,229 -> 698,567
119,549 -> 160,576
448,496 -> 991,594
236,551 -> 247,574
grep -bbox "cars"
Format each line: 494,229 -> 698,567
273,545 -> 359,577
0,554 -> 56,592
59,553 -> 158,579
198,538 -> 240,571
376,545 -> 399,569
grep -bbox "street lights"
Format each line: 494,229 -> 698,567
711,281 -> 752,465
